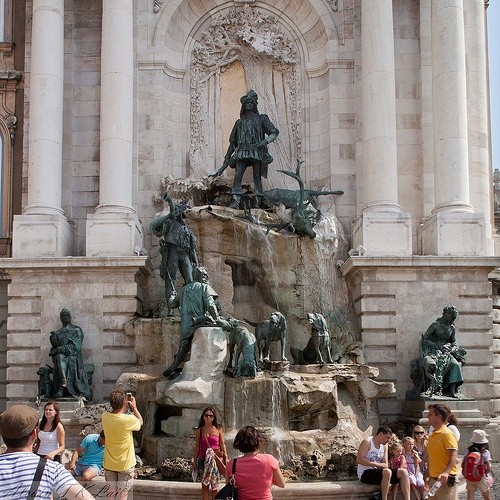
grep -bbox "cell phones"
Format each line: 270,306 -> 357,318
126,393 -> 131,402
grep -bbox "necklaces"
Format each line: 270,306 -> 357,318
207,426 -> 215,437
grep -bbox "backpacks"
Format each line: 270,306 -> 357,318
463,446 -> 487,482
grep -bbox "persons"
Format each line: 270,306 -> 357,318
356,404 -> 460,500
101,389 -> 143,500
35,401 -> 66,465
163,267 -> 220,377
410,305 -> 467,398
461,429 -> 494,500
0,405 -> 97,500
66,430 -> 106,481
192,407 -> 229,500
208,89 -> 280,209
151,192 -> 199,316
49,309 -> 93,401
226,425 -> 285,500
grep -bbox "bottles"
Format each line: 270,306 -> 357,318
428,481 -> 442,496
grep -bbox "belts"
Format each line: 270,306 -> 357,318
432,474 -> 456,481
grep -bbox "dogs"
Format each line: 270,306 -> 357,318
411,355 -> 438,398
243,311 -> 288,362
298,312 -> 334,365
35,368 -> 54,407
228,326 -> 260,377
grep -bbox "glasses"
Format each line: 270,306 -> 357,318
415,432 -> 423,435
204,414 -> 214,418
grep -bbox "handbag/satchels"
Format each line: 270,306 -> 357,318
32,438 -> 40,454
214,454 -> 226,474
195,456 -> 204,474
369,491 -> 382,500
215,460 -> 238,500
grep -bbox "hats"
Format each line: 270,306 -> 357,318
0,405 -> 38,439
471,429 -> 489,444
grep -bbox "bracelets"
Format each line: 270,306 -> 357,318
132,407 -> 137,411
223,455 -> 227,458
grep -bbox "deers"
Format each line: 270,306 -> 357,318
235,159 -> 344,240
